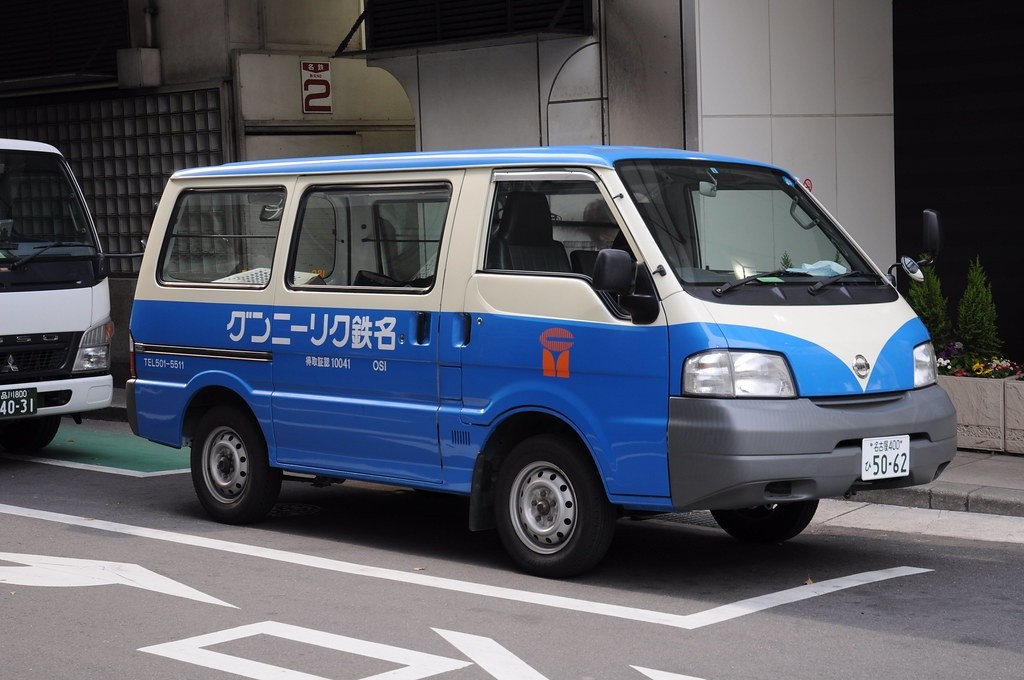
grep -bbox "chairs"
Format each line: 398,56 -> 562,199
489,189 -> 571,272
569,250 -> 600,275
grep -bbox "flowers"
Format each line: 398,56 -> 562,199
937,342 -> 1024,379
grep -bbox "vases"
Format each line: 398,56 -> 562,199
938,374 -> 1024,456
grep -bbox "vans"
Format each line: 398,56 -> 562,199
0,139 -> 149,453
124,147 -> 959,579
